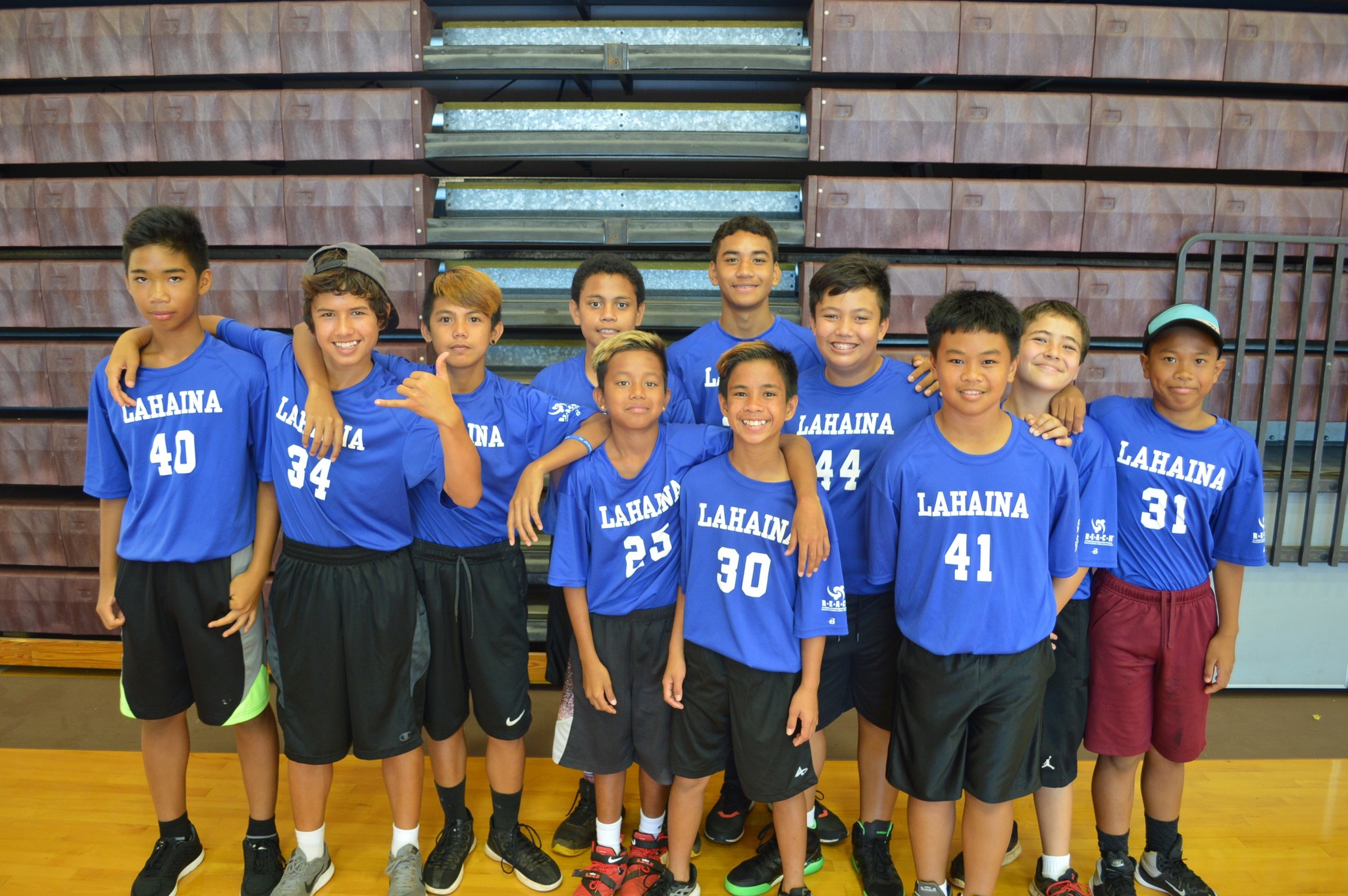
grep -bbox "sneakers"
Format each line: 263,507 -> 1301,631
949,820 -> 1022,889
768,789 -> 849,845
423,807 -> 477,895
130,823 -> 204,896
1088,850 -> 1137,896
551,777 -> 625,856
1028,856 -> 1092,896
912,880 -> 953,896
704,781 -> 755,843
270,842 -> 335,896
572,808 -> 703,896
384,843 -> 427,896
1134,832 -> 1219,896
484,813 -> 563,892
851,817 -> 905,896
725,818 -> 823,896
241,834 -> 287,896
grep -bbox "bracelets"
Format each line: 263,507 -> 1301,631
560,435 -> 594,457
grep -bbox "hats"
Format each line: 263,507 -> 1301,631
1142,302 -> 1225,359
304,242 -> 399,335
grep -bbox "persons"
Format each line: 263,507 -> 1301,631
79,203 -> 288,895
288,264 -> 614,894
722,249 -> 1074,895
1084,303 -> 1271,896
945,298 -> 1123,895
634,338 -> 853,894
543,330 -> 835,895
862,286 -> 1086,894
524,253 -> 705,860
652,215 -> 947,851
102,238 -> 486,895
